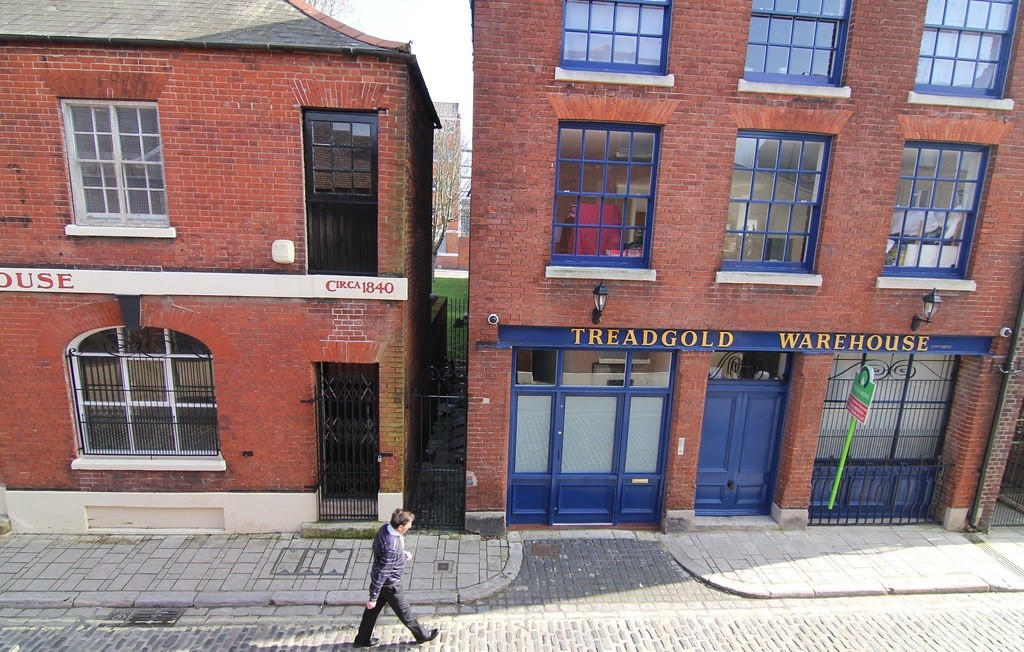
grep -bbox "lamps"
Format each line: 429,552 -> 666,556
911,287 -> 943,332
593,278 -> 609,324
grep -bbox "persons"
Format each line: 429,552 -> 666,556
355,507 -> 439,648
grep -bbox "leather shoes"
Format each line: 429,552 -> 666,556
416,629 -> 439,644
353,638 -> 379,648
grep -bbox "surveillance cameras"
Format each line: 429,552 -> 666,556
488,314 -> 499,324
999,327 -> 1012,337
